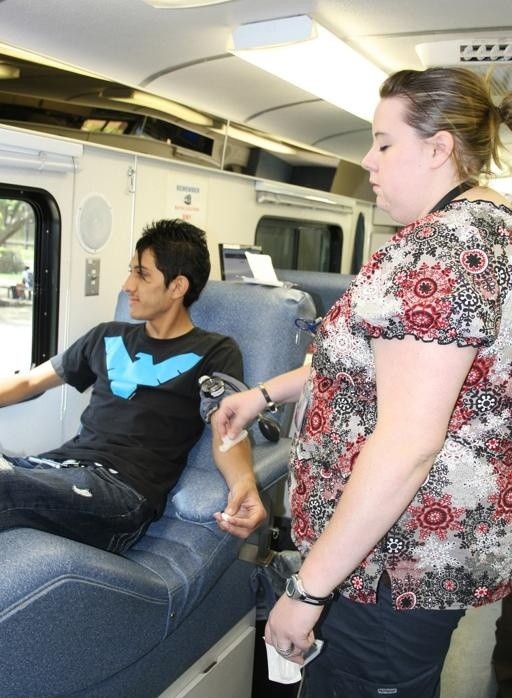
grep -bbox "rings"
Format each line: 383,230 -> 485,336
276,648 -> 292,656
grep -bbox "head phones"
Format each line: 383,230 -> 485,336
200,374 -> 281,444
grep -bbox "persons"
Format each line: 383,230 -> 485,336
0,218 -> 268,552
22,266 -> 32,299
217,67 -> 512,698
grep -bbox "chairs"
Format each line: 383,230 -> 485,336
255,269 -> 355,312
1,268 -> 329,698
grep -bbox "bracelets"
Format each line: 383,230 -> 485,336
257,381 -> 277,413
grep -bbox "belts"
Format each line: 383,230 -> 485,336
58,459 -> 121,480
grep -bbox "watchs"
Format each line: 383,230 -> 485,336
285,573 -> 334,606
